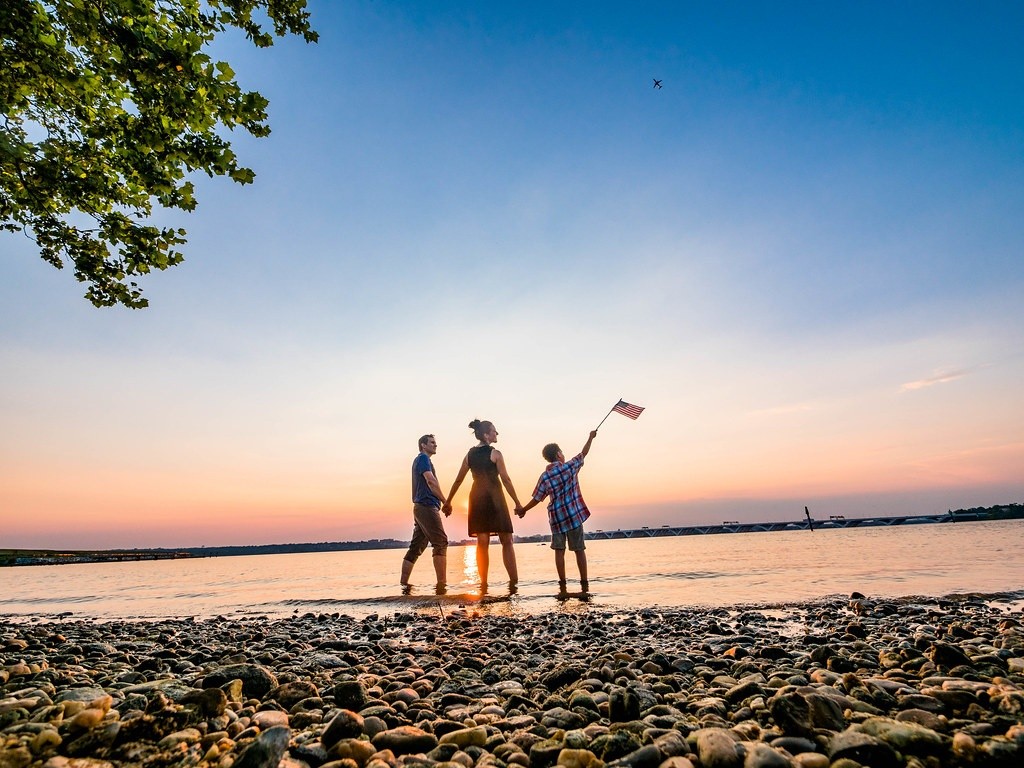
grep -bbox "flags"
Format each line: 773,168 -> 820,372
613,401 -> 645,420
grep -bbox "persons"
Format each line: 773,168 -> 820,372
515,430 -> 597,593
441,420 -> 525,581
396,434 -> 451,583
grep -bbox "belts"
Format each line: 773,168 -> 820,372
415,502 -> 440,506
